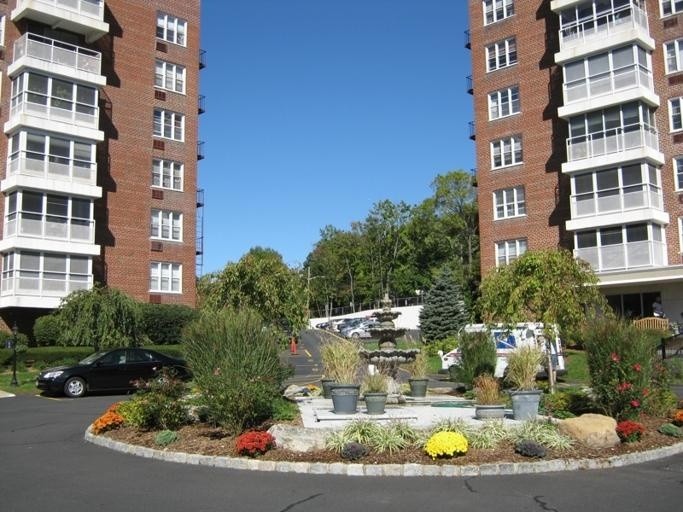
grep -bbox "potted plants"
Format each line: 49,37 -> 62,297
503,345 -> 544,421
408,347 -> 428,397
330,339 -> 361,415
469,371 -> 504,419
318,341 -> 334,398
358,365 -> 388,414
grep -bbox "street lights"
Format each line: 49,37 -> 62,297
8,319 -> 20,385
307,266 -> 326,318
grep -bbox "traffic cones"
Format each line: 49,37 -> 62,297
290,336 -> 296,352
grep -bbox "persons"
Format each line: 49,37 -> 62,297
652,296 -> 663,317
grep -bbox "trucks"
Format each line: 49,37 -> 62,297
435,321 -> 567,384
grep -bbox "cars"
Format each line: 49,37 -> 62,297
275,323 -> 298,345
33,344 -> 191,398
315,316 -> 381,340
